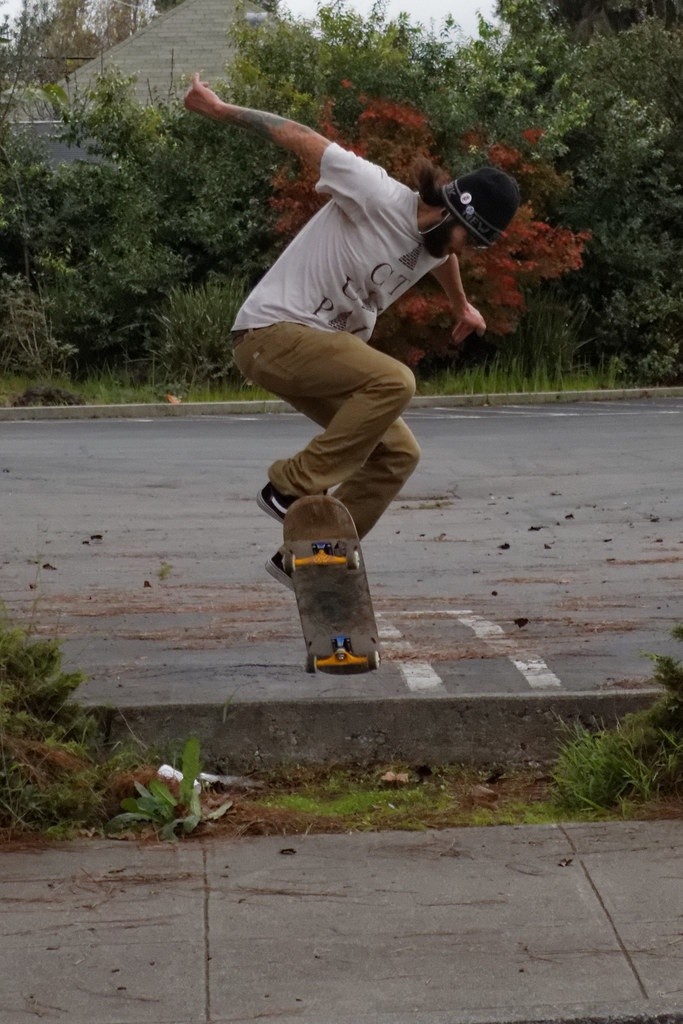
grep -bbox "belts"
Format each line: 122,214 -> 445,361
231,328 -> 261,343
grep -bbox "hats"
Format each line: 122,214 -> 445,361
441,167 -> 519,248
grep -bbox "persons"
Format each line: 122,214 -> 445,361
183,81 -> 520,592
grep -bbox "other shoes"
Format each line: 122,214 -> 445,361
265,545 -> 295,592
257,482 -> 327,523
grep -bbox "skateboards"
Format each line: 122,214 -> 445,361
280,493 -> 383,675
